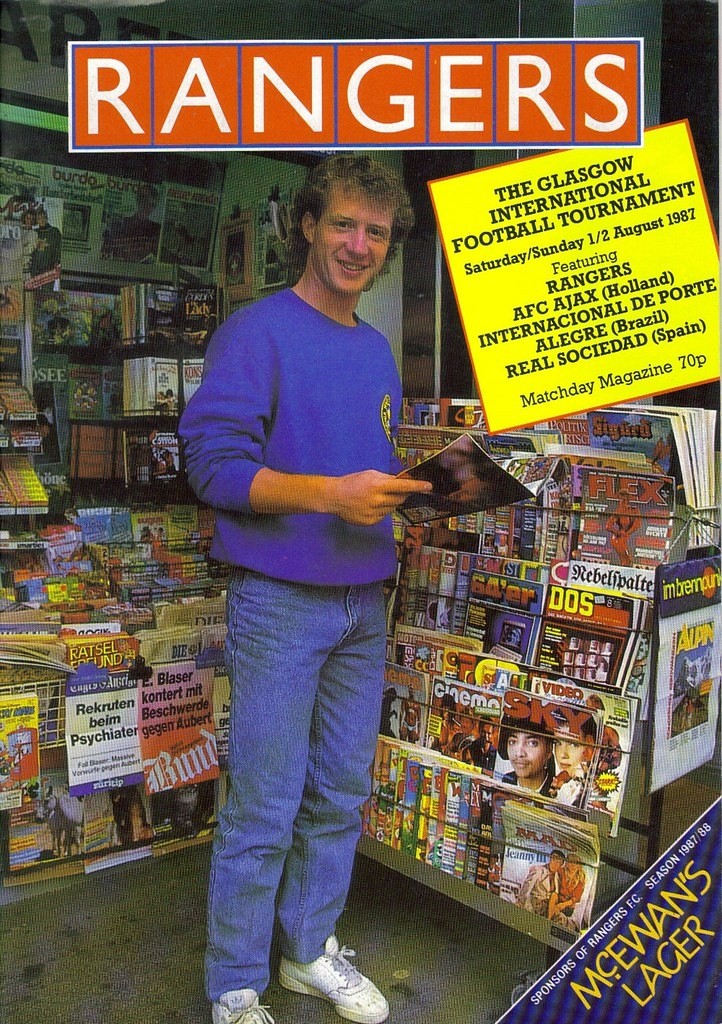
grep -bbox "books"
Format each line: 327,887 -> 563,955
390,431 -> 566,527
0,159 -> 722,932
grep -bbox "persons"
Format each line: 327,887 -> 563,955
606,488 -> 641,567
20,208 -> 38,289
547,721 -> 597,809
462,715 -> 497,779
430,694 -> 473,765
178,153 -> 434,1024
399,684 -> 421,743
518,850 -> 565,917
30,207 -> 61,291
530,853 -> 586,921
498,701 -> 558,799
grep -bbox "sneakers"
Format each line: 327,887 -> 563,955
278,936 -> 389,1024
212,988 -> 275,1024
